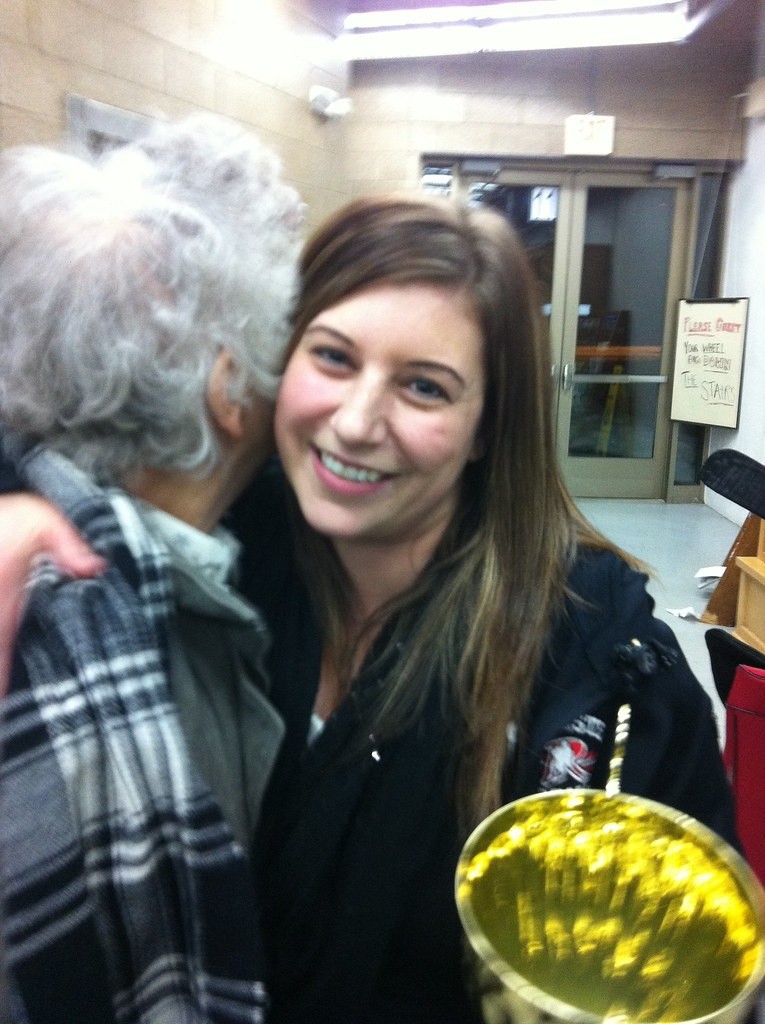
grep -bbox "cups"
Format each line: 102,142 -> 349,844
454,787 -> 765,1024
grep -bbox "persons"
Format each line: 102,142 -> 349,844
0,111 -> 307,1024
0,194 -> 758,1024
